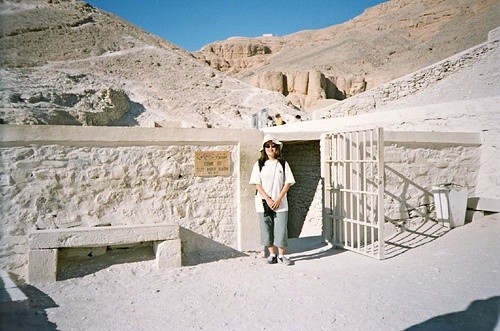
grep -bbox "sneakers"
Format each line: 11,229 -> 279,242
266,254 -> 278,263
277,256 -> 294,265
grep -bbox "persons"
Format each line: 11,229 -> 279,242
265,113 -> 303,127
248,134 -> 296,266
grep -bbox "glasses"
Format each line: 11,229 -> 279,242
264,143 -> 277,148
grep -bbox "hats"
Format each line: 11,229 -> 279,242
260,134 -> 283,152
